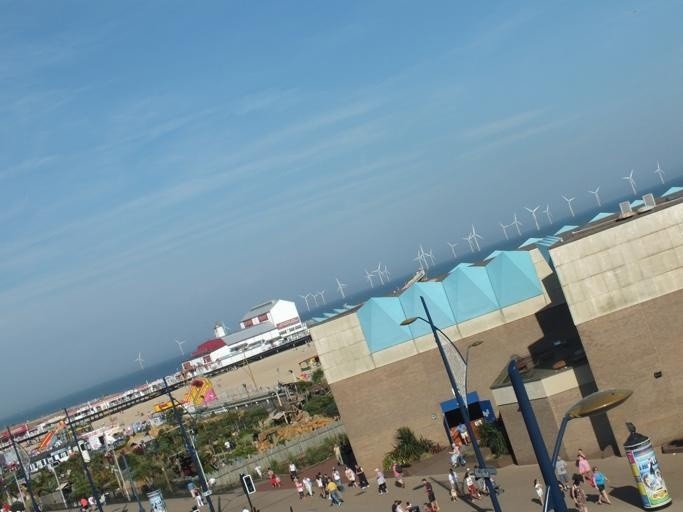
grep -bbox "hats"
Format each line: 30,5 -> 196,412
43,460 -> 63,471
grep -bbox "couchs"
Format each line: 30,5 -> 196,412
107,457 -> 114,465
242,475 -> 256,494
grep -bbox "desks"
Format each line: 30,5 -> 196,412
507,360 -> 633,512
45,464 -> 67,508
241,383 -> 252,404
61,406 -> 102,511
256,368 -> 288,425
288,368 -> 299,397
149,377 -> 214,511
399,295 -> 502,511
4,425 -> 41,511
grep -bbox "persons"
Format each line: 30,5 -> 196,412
225,440 -> 232,452
255,463 -> 261,477
187,482 -> 204,511
79,495 -> 105,511
252,431 -> 259,448
241,506 -> 249,512
